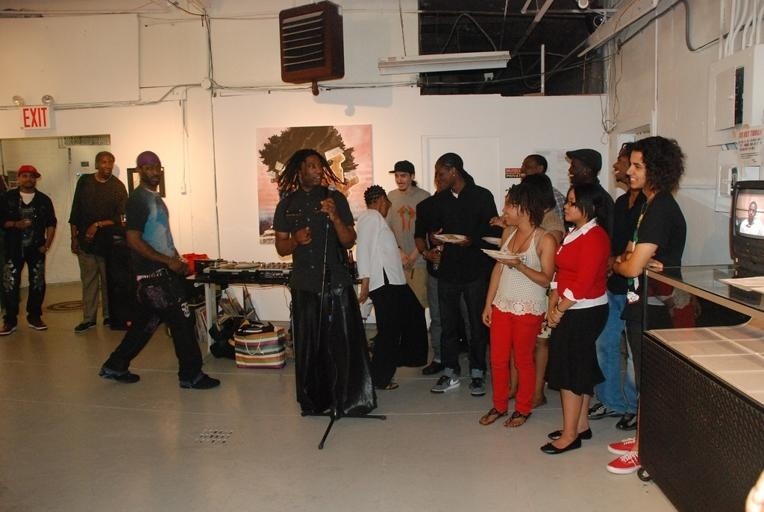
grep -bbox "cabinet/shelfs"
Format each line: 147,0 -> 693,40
637,265 -> 764,512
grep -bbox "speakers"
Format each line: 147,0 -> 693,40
102,223 -> 136,330
279,1 -> 345,84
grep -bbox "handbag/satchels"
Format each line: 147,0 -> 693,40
209,315 -> 245,358
232,320 -> 287,369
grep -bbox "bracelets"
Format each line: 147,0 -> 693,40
548,306 -> 565,324
43,245 -> 48,251
12,220 -> 16,230
94,222 -> 99,230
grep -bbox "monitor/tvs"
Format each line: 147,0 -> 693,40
729,180 -> 764,275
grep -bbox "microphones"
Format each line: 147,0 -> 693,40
327,183 -> 337,197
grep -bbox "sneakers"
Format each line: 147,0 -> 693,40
608,437 -> 636,455
587,401 -> 623,420
616,414 -> 636,430
74,321 -> 96,333
430,367 -> 461,393
469,368 -> 487,396
0,322 -> 17,336
26,316 -> 48,330
606,450 -> 640,474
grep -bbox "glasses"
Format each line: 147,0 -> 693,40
567,201 -> 575,207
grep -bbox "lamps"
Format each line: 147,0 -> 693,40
376,1 -> 511,76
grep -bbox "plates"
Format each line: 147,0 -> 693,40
431,232 -> 470,245
481,247 -> 525,262
482,236 -> 503,247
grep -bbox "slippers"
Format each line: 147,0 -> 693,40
503,411 -> 532,427
478,408 -> 508,425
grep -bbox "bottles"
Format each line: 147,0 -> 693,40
431,245 -> 444,271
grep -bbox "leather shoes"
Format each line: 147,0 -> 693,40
98,368 -> 140,383
540,437 -> 582,454
179,373 -> 220,390
374,383 -> 399,390
422,361 -> 440,375
548,427 -> 592,440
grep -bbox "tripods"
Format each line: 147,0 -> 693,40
301,216 -> 388,450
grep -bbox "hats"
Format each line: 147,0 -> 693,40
17,164 -> 41,178
566,149 -> 602,171
388,160 -> 415,173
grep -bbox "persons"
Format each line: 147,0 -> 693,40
488,172 -> 566,408
563,148 -> 616,243
539,182 -> 611,455
382,159 -> 433,312
738,201 -> 763,236
427,153 -> 502,398
606,134 -> 689,478
413,170 -> 441,377
586,139 -> 643,430
271,148 -> 379,415
66,150 -> 130,335
94,150 -> 222,390
518,154 -> 568,221
475,181 -> 558,429
354,184 -> 430,391
0,162 -> 58,338
